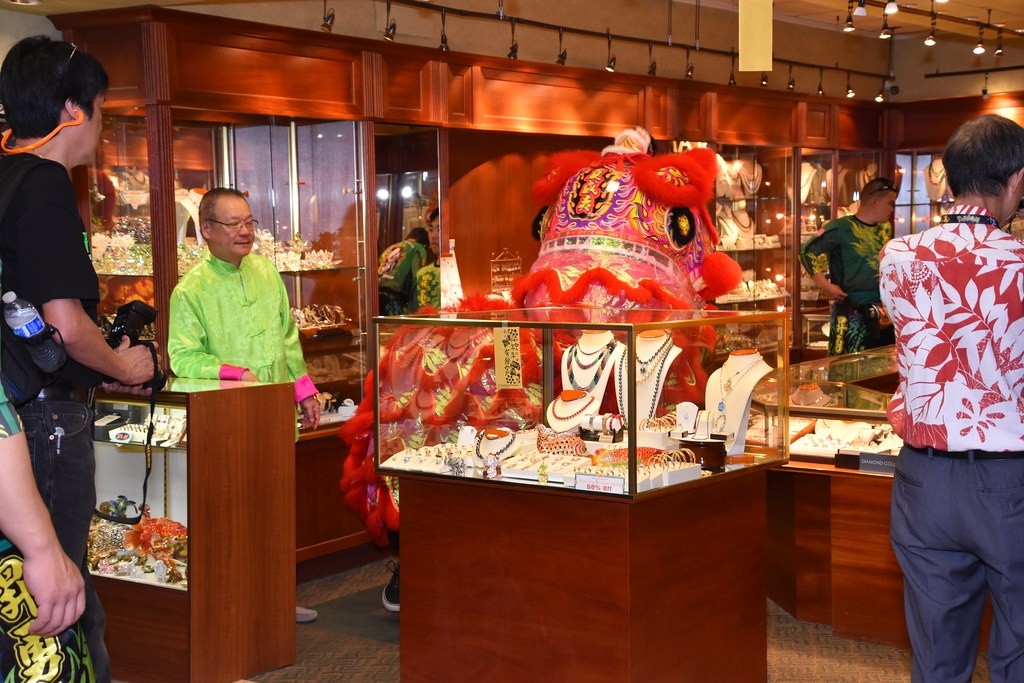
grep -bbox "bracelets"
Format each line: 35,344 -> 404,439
639,410 -> 678,433
291,304 -> 346,327
589,412 -> 599,433
602,413 -> 625,435
643,447 -> 696,475
714,415 -> 726,432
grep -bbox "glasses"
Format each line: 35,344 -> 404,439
868,184 -> 899,197
60,41 -> 81,69
205,218 -> 262,231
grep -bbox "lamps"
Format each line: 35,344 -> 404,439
973,27 -> 985,55
787,65 -> 796,91
878,10 -> 892,39
760,72 -> 768,87
981,73 -> 990,99
321,0 -> 334,32
383,0 -> 397,43
853,0 -> 867,15
884,0 -> 900,13
875,79 -> 887,102
439,7 -> 450,52
993,30 -> 1003,57
924,15 -> 937,46
842,0 -> 856,32
845,72 -> 855,98
556,27 -> 567,66
684,49 -> 694,80
606,36 -> 616,73
507,21 -> 518,61
646,42 -> 657,77
728,53 -> 738,86
817,68 -> 824,95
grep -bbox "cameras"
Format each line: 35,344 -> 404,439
109,300 -> 167,391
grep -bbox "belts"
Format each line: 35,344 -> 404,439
33,386 -> 93,403
902,440 -> 1024,461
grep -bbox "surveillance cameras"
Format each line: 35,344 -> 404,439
885,80 -> 900,95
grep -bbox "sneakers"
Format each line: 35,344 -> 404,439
382,560 -> 400,612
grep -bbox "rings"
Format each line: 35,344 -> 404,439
802,434 -> 843,449
509,451 -> 580,472
575,465 -> 641,478
393,441 -> 472,468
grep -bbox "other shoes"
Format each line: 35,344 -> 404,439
296,606 -> 318,622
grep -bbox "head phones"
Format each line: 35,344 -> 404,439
1,108 -> 84,154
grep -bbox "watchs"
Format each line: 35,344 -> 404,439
336,398 -> 359,412
314,391 -> 333,412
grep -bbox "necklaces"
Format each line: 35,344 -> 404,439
567,338 -> 619,394
691,410 -> 711,440
680,403 -> 696,420
739,160 -> 763,193
619,335 -> 674,428
415,328 -> 493,411
552,395 -> 597,420
801,168 -> 816,189
839,166 -> 845,172
732,210 -> 753,233
127,171 -> 146,186
718,354 -> 766,415
718,215 -> 737,234
389,324 -> 437,401
186,194 -> 200,210
929,163 -> 947,185
862,169 -> 879,186
475,430 -> 516,460
791,393 -> 827,406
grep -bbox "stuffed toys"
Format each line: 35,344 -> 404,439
338,127 -> 742,542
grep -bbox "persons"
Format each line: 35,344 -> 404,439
381,563 -> 402,611
800,174 -> 896,357
168,187 -> 322,624
0,33 -> 162,683
1001,194 -> 1024,240
879,113 -> 1024,683
0,382 -> 98,683
377,228 -> 430,317
406,205 -> 442,312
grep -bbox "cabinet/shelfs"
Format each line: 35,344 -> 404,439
58,117 -> 1024,683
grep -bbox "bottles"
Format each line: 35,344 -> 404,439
2,290 -> 66,372
